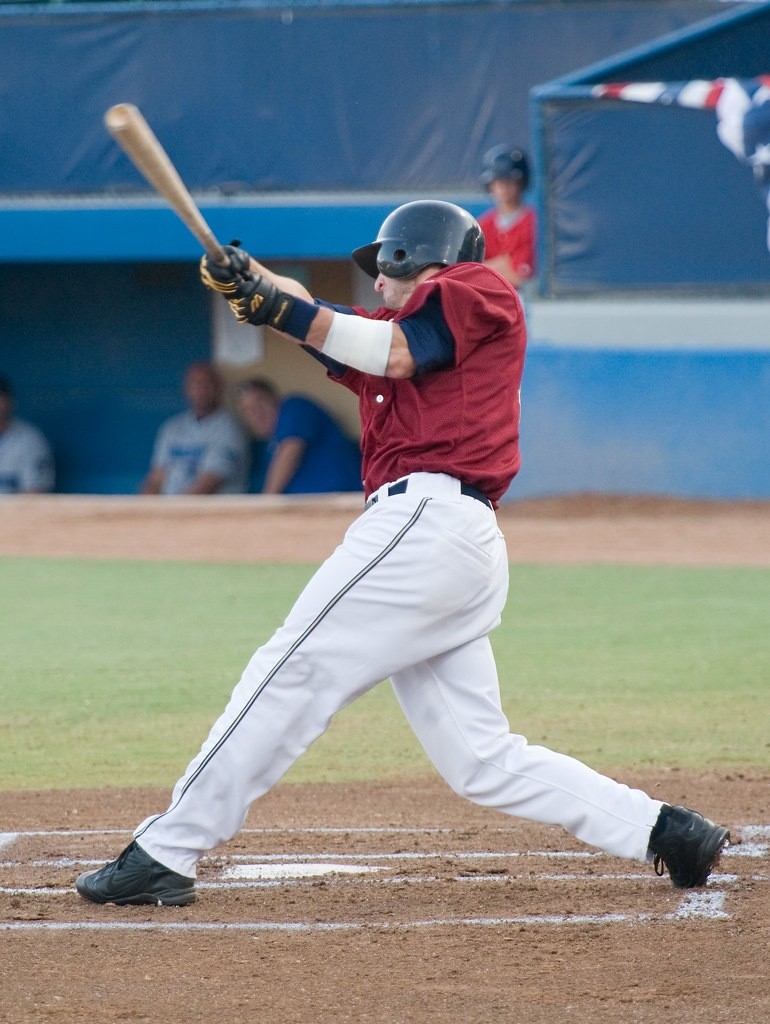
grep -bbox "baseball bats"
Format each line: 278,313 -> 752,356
102,103 -> 234,266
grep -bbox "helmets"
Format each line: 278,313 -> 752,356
480,143 -> 532,186
352,199 -> 486,280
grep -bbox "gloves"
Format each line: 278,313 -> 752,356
223,269 -> 296,332
200,246 -> 258,298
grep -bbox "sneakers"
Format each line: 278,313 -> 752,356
76,840 -> 198,907
648,803 -> 732,890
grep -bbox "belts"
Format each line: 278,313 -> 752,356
364,475 -> 491,511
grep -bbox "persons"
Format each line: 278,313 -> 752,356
442,143 -> 538,302
79,200 -> 729,905
0,369 -> 55,494
140,364 -> 366,493
